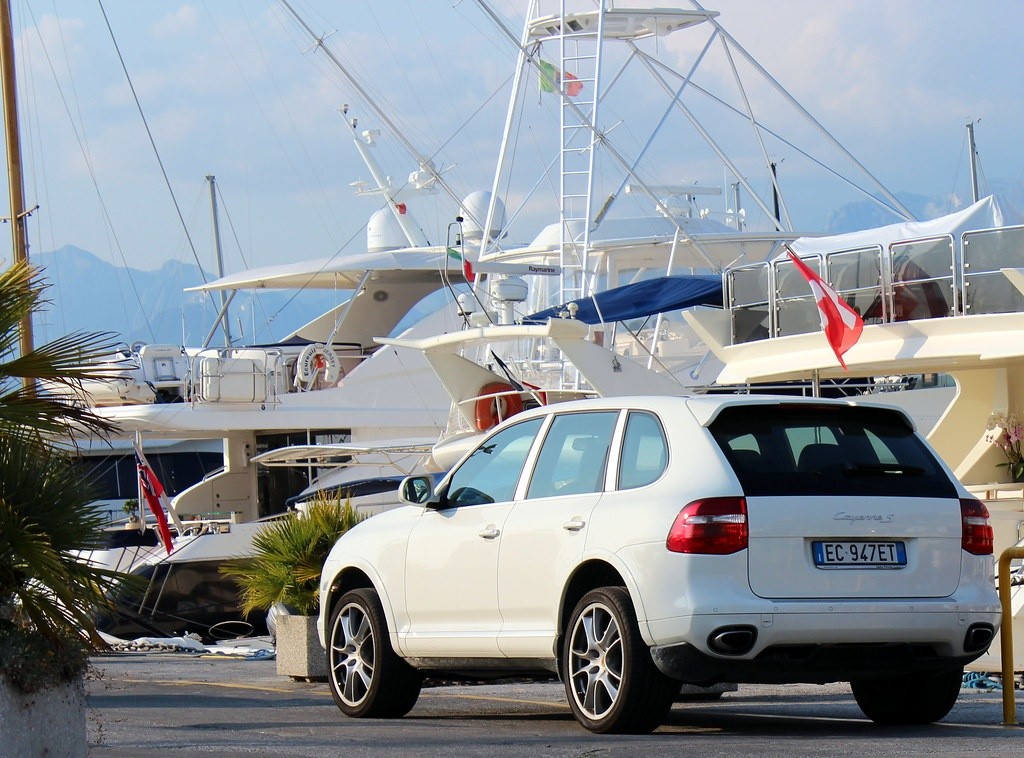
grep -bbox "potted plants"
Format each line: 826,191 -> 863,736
217,484 -> 374,684
122,498 -> 140,530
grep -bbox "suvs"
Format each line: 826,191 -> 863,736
318,396 -> 1003,736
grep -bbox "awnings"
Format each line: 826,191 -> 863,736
523,274 -> 722,324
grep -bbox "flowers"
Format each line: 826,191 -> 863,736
984,410 -> 1024,479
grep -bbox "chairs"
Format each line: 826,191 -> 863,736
731,448 -> 770,490
797,444 -> 848,479
558,445 -> 632,491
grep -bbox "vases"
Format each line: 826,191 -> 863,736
1012,466 -> 1024,483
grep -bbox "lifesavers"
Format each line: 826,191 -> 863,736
474,382 -> 522,432
297,343 -> 339,383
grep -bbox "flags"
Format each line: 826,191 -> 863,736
135,451 -> 174,556
787,253 -> 864,372
540,60 -> 583,97
866,256 -> 950,317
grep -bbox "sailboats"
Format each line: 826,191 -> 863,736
1,2 -> 1024,657
